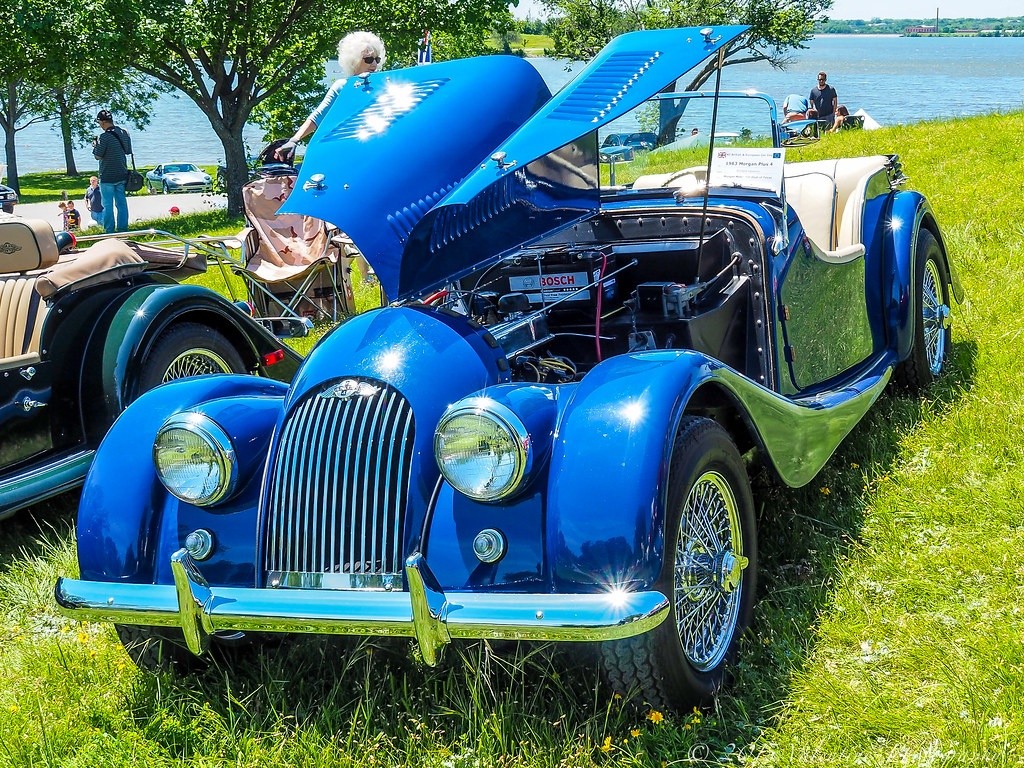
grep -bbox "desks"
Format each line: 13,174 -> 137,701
708,132 -> 740,144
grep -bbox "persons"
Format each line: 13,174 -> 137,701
58,201 -> 81,231
83,110 -> 132,234
782,72 -> 849,133
690,128 -> 698,136
274,30 -> 385,287
168,207 -> 180,217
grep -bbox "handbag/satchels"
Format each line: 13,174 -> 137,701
125,170 -> 144,191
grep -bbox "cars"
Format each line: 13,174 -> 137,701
52,24 -> 965,722
0,184 -> 20,213
600,131 -> 659,163
1,219 -> 314,517
145,162 -> 214,196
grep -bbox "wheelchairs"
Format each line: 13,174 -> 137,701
196,138 -> 361,340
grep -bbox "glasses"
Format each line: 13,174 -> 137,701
363,56 -> 381,64
817,79 -> 827,80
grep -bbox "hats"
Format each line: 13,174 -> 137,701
169,207 -> 180,212
97,110 -> 112,123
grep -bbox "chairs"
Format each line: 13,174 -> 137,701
837,116 -> 865,130
0,219 -> 149,370
196,172 -> 355,324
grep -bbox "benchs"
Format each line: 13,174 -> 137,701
638,153 -> 909,395
